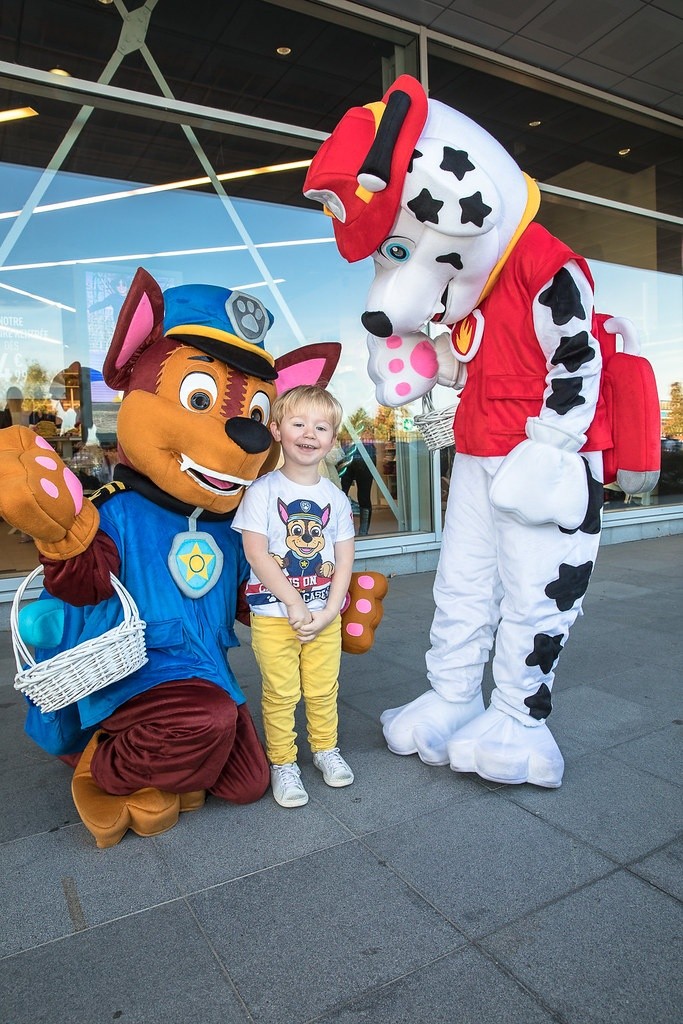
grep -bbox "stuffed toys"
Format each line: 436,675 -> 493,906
0,267 -> 387,850
304,74 -> 660,789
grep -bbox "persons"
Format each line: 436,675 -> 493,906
0,387 -> 62,429
336,418 -> 377,537
229,385 -> 355,808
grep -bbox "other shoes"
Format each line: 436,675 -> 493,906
19,534 -> 33,542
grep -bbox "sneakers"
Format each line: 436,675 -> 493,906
313,747 -> 354,788
269,762 -> 309,808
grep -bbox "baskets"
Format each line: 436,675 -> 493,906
10,564 -> 150,714
414,389 -> 460,450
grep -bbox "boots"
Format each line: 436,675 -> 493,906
357,509 -> 371,535
